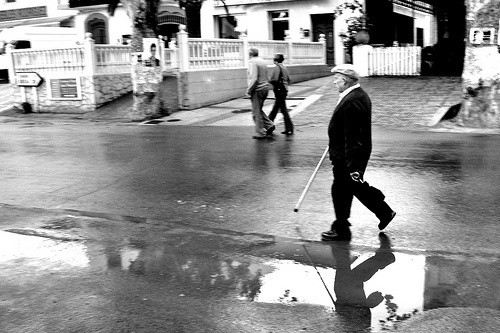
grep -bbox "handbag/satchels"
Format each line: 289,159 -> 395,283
273,64 -> 287,97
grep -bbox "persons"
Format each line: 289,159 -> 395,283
320,63 -> 396,241
245,47 -> 295,140
144,42 -> 160,68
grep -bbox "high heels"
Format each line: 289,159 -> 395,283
281,127 -> 294,134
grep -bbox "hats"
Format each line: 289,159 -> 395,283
330,64 -> 359,79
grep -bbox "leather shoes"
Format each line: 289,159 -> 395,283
252,126 -> 275,139
378,209 -> 396,230
321,230 -> 352,242
